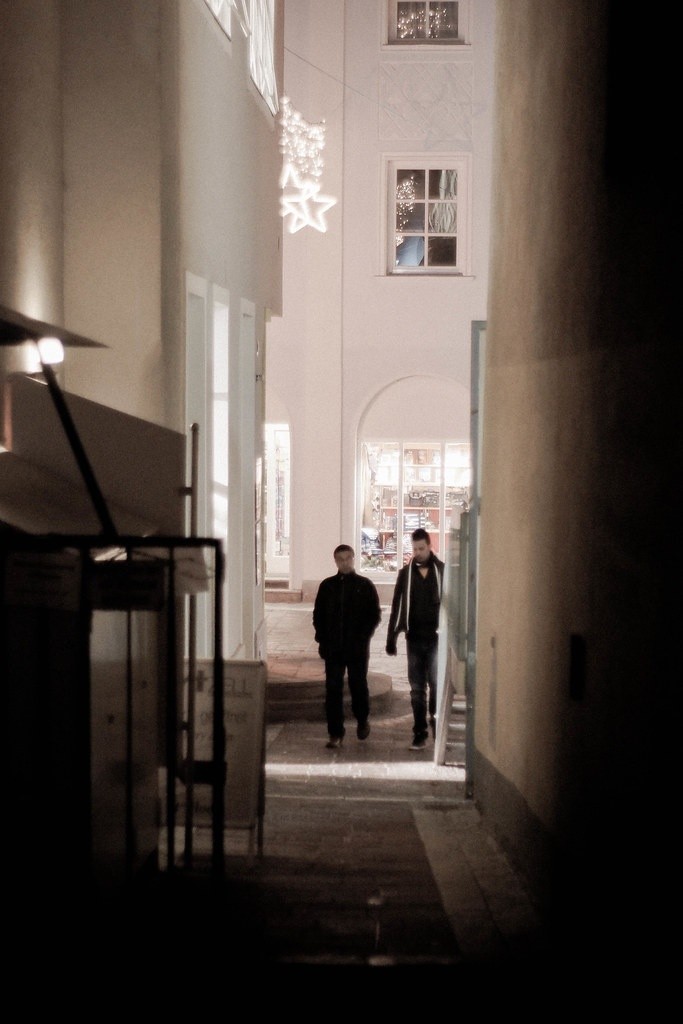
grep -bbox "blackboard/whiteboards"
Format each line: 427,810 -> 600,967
155,658 -> 268,828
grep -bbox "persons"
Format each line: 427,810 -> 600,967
385,529 -> 445,749
313,544 -> 382,748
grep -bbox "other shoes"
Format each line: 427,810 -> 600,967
326,729 -> 347,748
428,717 -> 438,739
408,736 -> 428,752
356,719 -> 371,740
